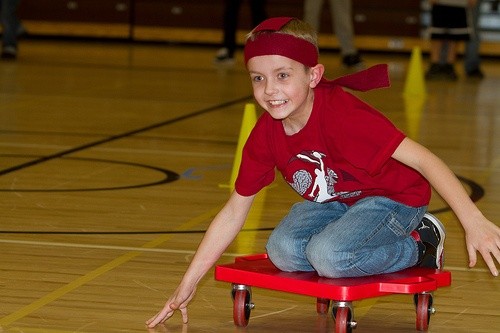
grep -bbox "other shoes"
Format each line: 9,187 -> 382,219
445,64 -> 457,80
344,55 -> 361,66
426,64 -> 440,79
216,50 -> 234,59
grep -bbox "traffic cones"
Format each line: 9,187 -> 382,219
232,103 -> 261,185
404,48 -> 429,95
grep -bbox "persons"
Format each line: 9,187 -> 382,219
213,0 -> 488,83
146,16 -> 500,332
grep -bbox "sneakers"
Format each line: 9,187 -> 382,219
414,211 -> 446,270
